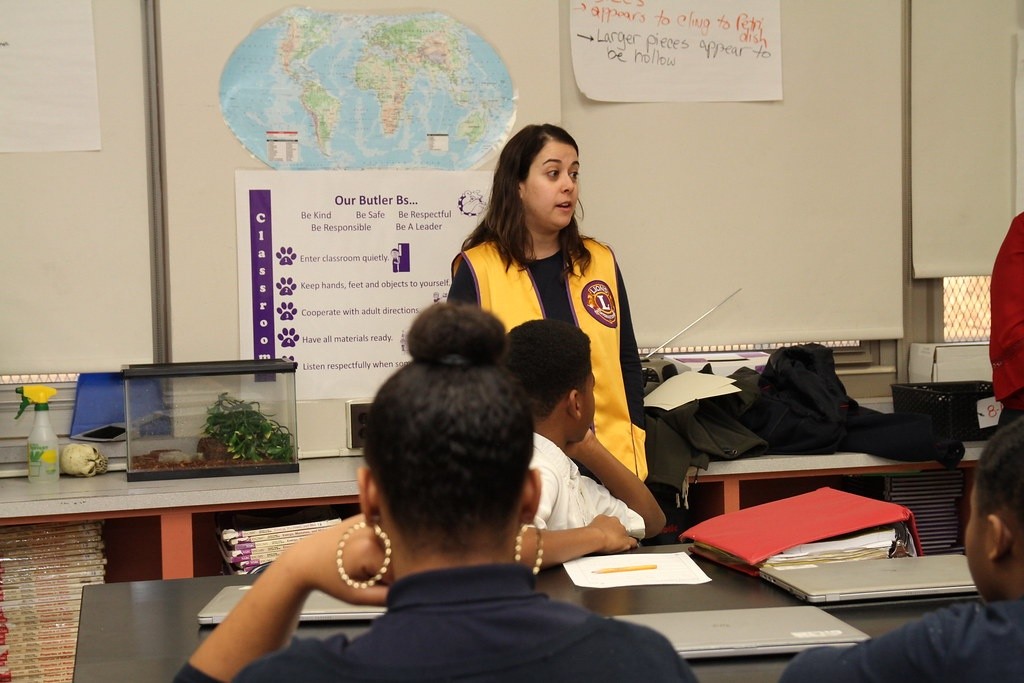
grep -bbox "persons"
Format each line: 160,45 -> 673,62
779,211 -> 1024,683
446,124 -> 669,570
175,302 -> 703,683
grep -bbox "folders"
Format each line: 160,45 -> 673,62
681,485 -> 923,580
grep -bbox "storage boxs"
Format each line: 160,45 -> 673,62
663,351 -> 770,376
119,358 -> 299,483
908,342 -> 992,382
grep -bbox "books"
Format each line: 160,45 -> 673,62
0,519 -> 108,683
782,527 -> 896,556
214,514 -> 343,576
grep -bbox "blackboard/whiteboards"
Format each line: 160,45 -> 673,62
0,0 -> 908,377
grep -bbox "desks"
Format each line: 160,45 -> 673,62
73,542 -> 977,683
0,441 -> 986,580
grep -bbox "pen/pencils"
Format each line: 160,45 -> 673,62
591,565 -> 657,573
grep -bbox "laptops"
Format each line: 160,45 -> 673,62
760,554 -> 979,603
197,585 -> 387,625
610,606 -> 871,659
